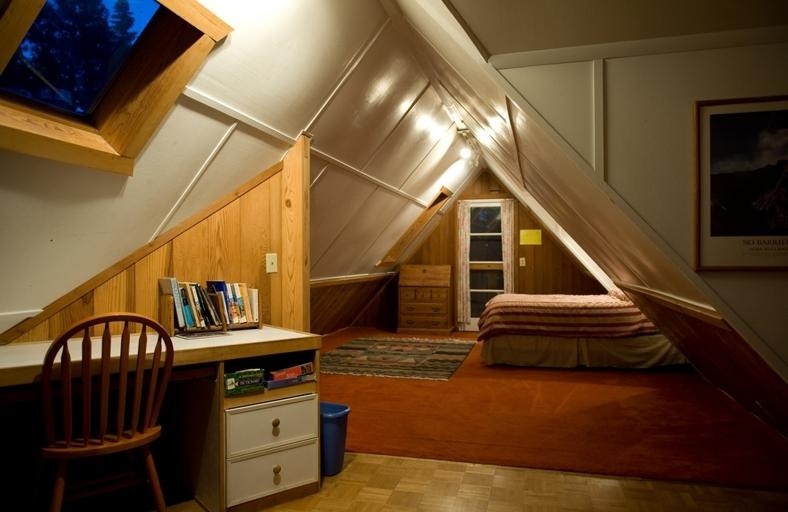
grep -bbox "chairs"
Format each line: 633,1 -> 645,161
40,312 -> 173,512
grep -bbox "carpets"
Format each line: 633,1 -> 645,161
320,337 -> 476,381
320,325 -> 788,488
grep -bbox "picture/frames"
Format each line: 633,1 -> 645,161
692,93 -> 788,272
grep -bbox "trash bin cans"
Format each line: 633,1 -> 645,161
320,402 -> 351,476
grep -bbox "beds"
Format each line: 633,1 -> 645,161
477,294 -> 691,369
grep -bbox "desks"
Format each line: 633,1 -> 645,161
0,323 -> 322,512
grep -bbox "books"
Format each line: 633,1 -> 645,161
159,277 -> 261,340
